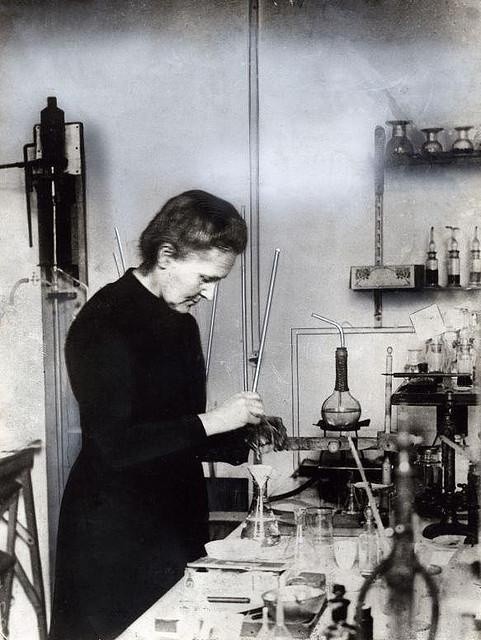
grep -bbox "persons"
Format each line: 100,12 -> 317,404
50,188 -> 289,640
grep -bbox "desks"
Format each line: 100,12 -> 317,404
0,441 -> 50,640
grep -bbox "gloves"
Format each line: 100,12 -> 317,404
243,416 -> 289,452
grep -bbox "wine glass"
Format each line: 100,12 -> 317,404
386,118 -> 475,158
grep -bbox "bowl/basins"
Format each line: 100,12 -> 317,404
259,585 -> 329,622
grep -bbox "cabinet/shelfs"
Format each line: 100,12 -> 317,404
371,148 -> 481,290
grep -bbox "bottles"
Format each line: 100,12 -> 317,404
394,329 -> 476,392
282,510 -> 318,568
321,343 -> 368,425
419,223 -> 481,290
238,465 -> 279,547
354,504 -> 390,573
